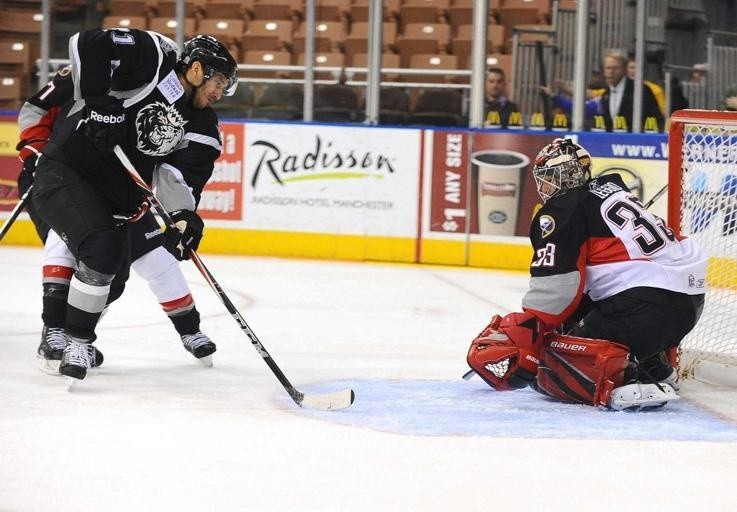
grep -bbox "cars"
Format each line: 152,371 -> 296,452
469,149 -> 532,236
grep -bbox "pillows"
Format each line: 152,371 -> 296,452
181,35 -> 239,97
533,138 -> 591,202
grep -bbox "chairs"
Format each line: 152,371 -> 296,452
0,0 -> 593,132
646,357 -> 674,382
181,331 -> 216,358
38,327 -> 103,380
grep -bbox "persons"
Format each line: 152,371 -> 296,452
484,66 -> 523,125
585,48 -> 665,132
535,47 -> 668,132
497,137 -> 705,410
722,95 -> 737,111
16,59 -> 217,362
28,25 -> 239,381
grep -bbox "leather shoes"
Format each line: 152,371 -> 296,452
83,96 -> 128,157
162,209 -> 204,261
